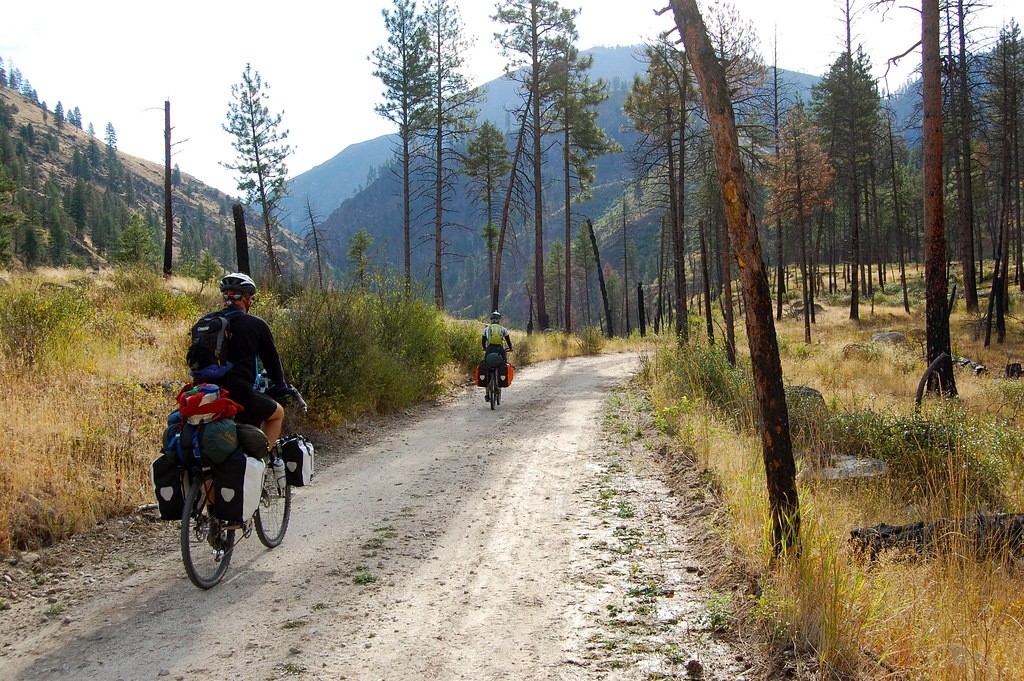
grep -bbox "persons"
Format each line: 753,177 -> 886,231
481,312 -> 513,401
192,272 -> 288,465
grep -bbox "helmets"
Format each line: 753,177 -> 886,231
220,273 -> 258,295
490,312 -> 500,323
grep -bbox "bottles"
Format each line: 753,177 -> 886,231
274,457 -> 287,487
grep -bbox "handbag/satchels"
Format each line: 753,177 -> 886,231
473,360 -> 490,387
498,361 -> 513,387
149,383 -> 269,524
279,433 -> 315,486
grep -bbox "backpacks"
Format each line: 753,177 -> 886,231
488,324 -> 502,345
186,310 -> 245,376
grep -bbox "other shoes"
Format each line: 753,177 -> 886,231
485,395 -> 490,402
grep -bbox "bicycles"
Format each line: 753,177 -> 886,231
479,345 -> 514,410
182,372 -> 308,591
